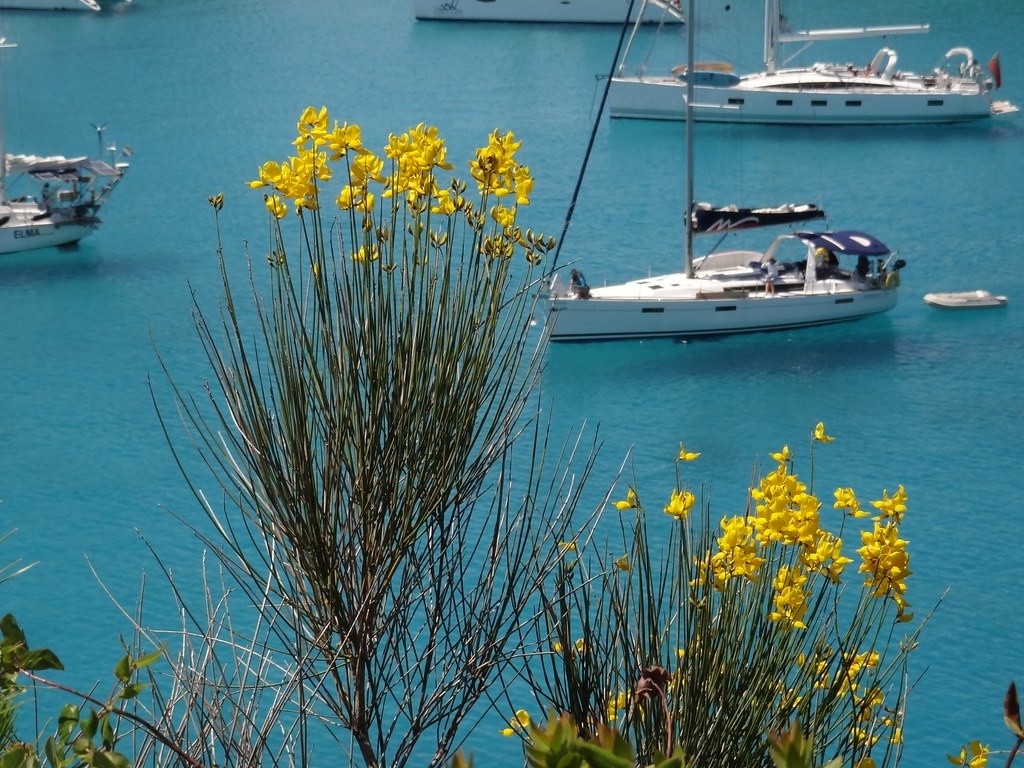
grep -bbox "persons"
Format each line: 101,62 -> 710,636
761,258 -> 779,295
42,183 -> 51,214
568,268 -> 591,299
817,246 -> 840,279
855,255 -> 869,283
873,259 -> 888,282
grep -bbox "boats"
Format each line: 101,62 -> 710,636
926,291 -> 1007,313
2,119 -> 133,258
593,0 -> 998,121
419,1 -> 683,25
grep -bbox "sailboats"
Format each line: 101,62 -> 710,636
534,3 -> 904,342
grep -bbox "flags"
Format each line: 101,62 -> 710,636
988,52 -> 1001,91
122,147 -> 133,157
